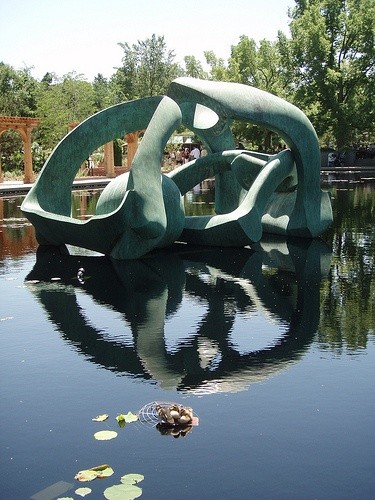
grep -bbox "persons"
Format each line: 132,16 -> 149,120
275,143 -> 287,154
174,145 -> 202,171
